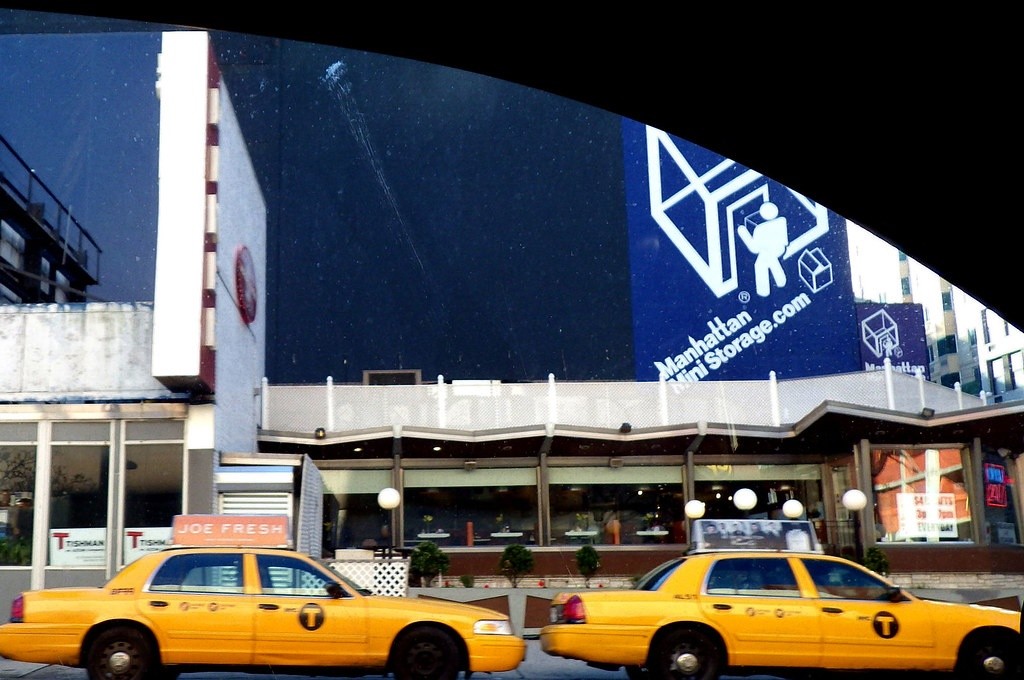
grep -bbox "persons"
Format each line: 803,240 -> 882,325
703,522 -> 814,550
376,524 -> 392,546
0,491 -> 33,544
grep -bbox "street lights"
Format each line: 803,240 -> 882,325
378,486 -> 401,558
844,491 -> 868,568
734,488 -> 756,517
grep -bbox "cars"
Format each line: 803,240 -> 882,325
537,518 -> 1021,680
0,514 -> 527,680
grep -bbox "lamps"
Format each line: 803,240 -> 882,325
920,407 -> 935,420
619,422 -> 632,434
315,428 -> 326,440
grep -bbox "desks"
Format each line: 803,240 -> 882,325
629,530 -> 669,544
490,532 -> 522,545
565,531 -> 599,545
417,533 -> 450,545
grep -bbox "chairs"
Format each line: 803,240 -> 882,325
237,563 -> 272,588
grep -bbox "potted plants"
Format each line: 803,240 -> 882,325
576,513 -> 589,531
423,515 -> 433,534
496,514 -> 503,532
632,513 -> 658,531
0,449 -> 35,506
